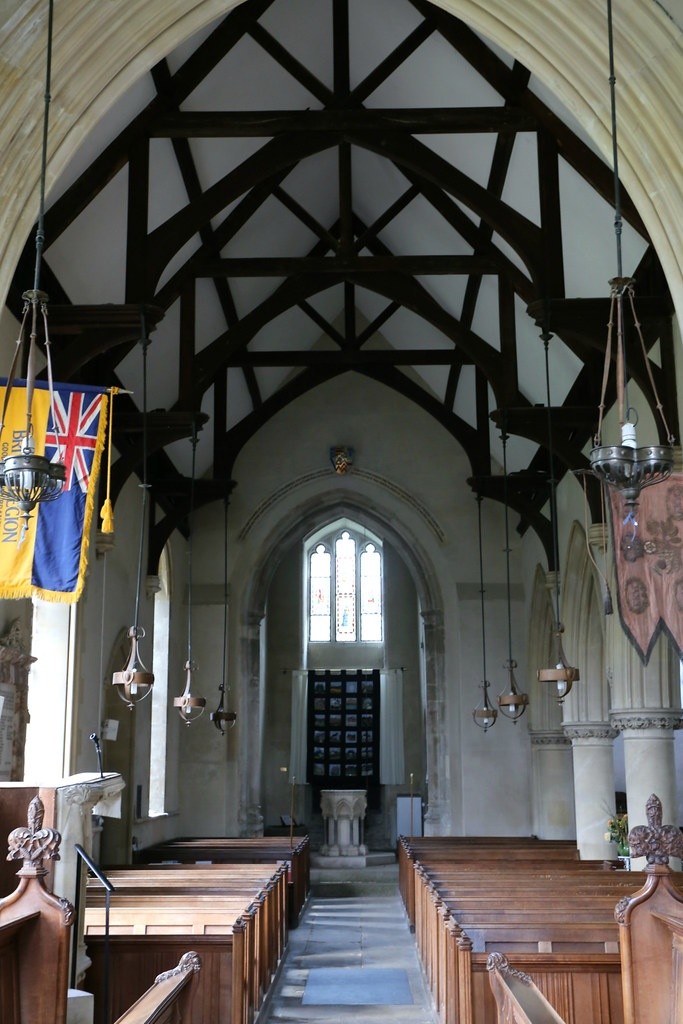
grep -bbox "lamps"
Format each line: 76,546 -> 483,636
498,423 -> 528,724
588,0 -> 674,512
171,420 -> 207,725
472,491 -> 498,733
537,326 -> 580,708
0,0 -> 73,531
209,490 -> 238,735
110,311 -> 156,710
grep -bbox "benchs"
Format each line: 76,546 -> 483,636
72,836 -> 312,1024
397,829 -> 681,1024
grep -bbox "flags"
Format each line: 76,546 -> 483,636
0,386 -> 109,603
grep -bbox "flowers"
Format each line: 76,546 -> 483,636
600,799 -> 631,843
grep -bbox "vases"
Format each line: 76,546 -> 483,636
617,842 -> 630,857
